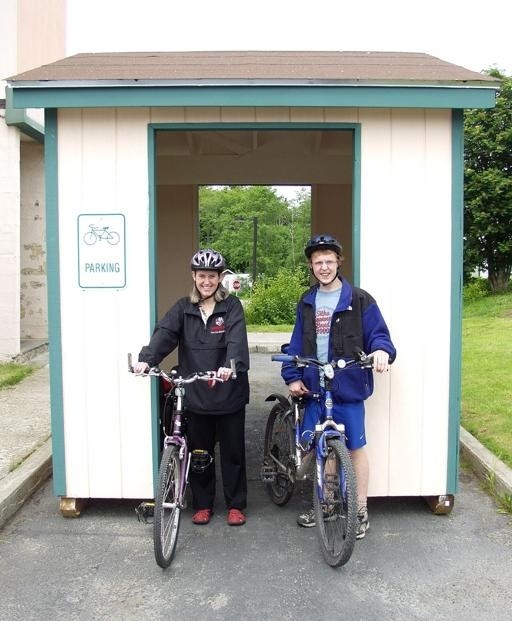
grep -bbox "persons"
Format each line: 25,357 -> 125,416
132,247 -> 251,527
280,233 -> 397,540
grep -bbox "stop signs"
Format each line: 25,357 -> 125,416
232,280 -> 241,291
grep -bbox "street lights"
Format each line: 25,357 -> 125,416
234,216 -> 257,286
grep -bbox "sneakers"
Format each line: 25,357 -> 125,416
295,499 -> 339,527
191,506 -> 215,526
341,511 -> 371,542
224,507 -> 246,528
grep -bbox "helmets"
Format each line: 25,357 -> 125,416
191,247 -> 225,274
304,232 -> 343,260
